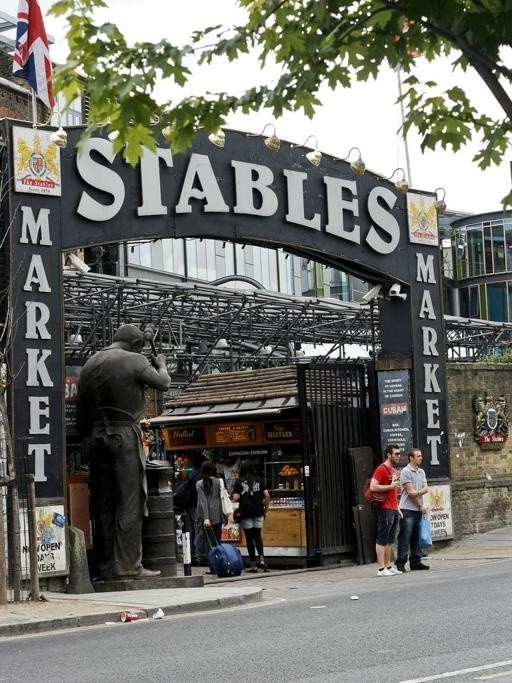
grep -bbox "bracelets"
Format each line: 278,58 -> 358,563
418,504 -> 423,507
418,491 -> 419,495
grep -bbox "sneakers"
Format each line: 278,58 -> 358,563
244,565 -> 258,573
396,564 -> 408,574
259,560 -> 269,571
376,566 -> 396,576
409,561 -> 430,571
388,567 -> 403,574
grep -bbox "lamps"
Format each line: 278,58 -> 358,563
108,109 -> 225,149
332,146 -> 365,177
290,134 -> 322,167
377,167 -> 409,194
431,187 -> 447,212
246,123 -> 281,153
32,110 -> 67,149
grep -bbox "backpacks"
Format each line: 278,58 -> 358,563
362,477 -> 387,507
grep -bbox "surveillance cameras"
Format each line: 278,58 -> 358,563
362,284 -> 384,302
68,253 -> 92,274
389,284 -> 407,301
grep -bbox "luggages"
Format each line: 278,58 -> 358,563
208,542 -> 244,577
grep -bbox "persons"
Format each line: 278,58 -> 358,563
74,322 -> 172,582
393,447 -> 430,574
369,444 -> 404,577
181,456 -> 271,576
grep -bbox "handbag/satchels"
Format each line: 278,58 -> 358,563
218,489 -> 234,518
416,514 -> 433,549
